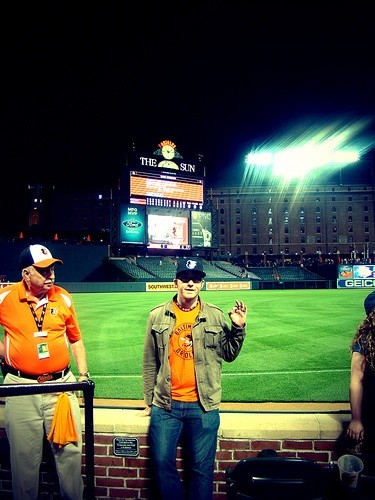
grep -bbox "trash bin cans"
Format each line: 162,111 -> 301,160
226,449 -> 340,500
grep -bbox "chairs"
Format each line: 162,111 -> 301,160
225,456 -> 326,500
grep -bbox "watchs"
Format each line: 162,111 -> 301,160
78,371 -> 90,378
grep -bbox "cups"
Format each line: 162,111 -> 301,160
337,454 -> 364,487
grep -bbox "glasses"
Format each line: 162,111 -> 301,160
176,275 -> 204,283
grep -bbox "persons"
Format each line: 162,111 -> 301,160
0,244 -> 91,500
346,291 -> 375,477
198,223 -> 212,247
142,259 -> 248,500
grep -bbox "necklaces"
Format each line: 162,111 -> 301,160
175,300 -> 199,312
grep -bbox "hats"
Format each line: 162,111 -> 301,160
19,244 -> 63,268
176,259 -> 206,278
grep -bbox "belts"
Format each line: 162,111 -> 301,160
7,365 -> 71,381
